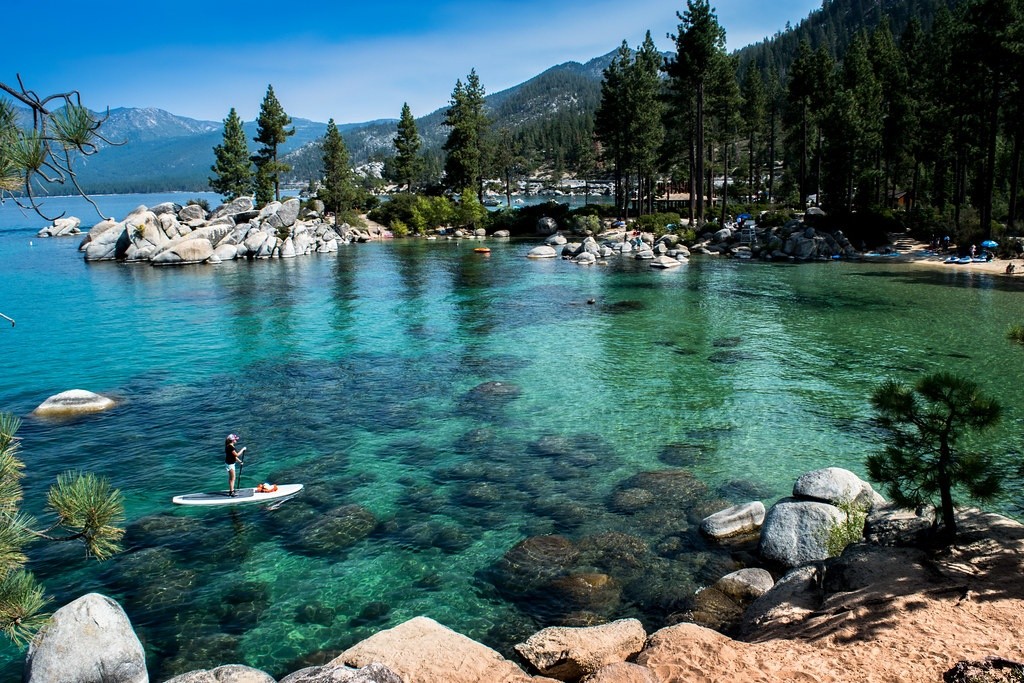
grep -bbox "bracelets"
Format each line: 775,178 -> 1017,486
240,462 -> 242,463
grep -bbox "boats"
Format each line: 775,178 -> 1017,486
482,197 -> 503,206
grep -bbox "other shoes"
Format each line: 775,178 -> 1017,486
230,492 -> 239,498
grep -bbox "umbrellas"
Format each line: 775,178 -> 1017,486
737,213 -> 751,220
981,240 -> 999,248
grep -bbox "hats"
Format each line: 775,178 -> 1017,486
227,434 -> 239,440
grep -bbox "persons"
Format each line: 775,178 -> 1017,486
986,253 -> 995,262
728,215 -> 733,226
741,210 -> 744,214
969,245 -> 976,258
224,434 -> 246,497
636,224 -> 640,235
1006,263 -> 1015,273
637,237 -> 642,251
747,208 -> 751,214
713,216 -> 721,225
936,235 -> 950,251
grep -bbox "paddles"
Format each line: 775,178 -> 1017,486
237,450 -> 246,489
